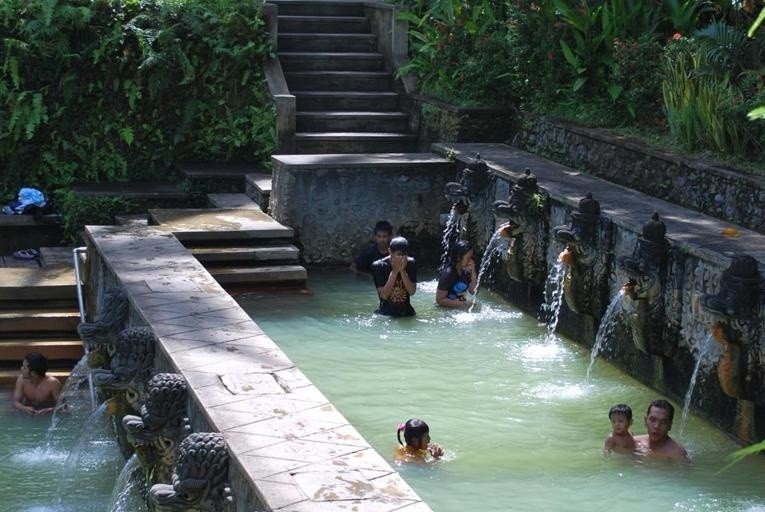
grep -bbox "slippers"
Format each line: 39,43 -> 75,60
13,249 -> 38,260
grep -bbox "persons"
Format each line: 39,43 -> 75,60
435,239 -> 483,313
631,398 -> 691,462
601,403 -> 639,461
9,351 -> 69,416
349,219 -> 394,281
391,415 -> 447,470
370,235 -> 419,318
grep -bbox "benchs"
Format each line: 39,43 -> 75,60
0,213 -> 64,226
